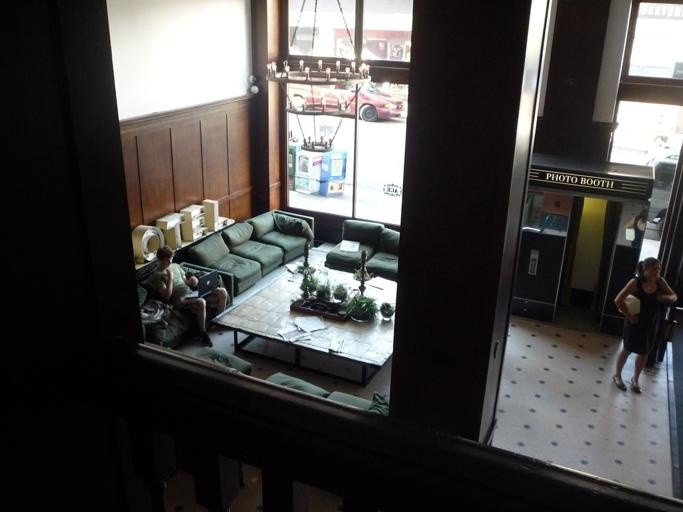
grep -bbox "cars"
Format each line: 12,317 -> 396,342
287,79 -> 405,121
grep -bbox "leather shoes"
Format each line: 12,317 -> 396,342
631,377 -> 642,393
613,376 -> 627,390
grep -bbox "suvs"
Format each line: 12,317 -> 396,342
647,154 -> 680,240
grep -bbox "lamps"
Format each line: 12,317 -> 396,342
249,75 -> 261,96
265,0 -> 373,152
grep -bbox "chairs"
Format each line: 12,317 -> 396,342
261,371 -> 389,419
193,344 -> 253,374
325,219 -> 400,281
189,211 -> 314,296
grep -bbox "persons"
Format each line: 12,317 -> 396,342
623,201 -> 649,249
612,257 -> 678,393
151,244 -> 227,347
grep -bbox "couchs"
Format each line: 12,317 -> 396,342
135,261 -> 234,345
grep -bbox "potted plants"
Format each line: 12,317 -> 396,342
350,298 -> 378,323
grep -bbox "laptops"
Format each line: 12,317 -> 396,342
185,270 -> 217,299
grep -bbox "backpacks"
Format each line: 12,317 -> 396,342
139,299 -> 173,324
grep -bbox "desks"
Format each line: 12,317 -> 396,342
211,258 -> 399,388
